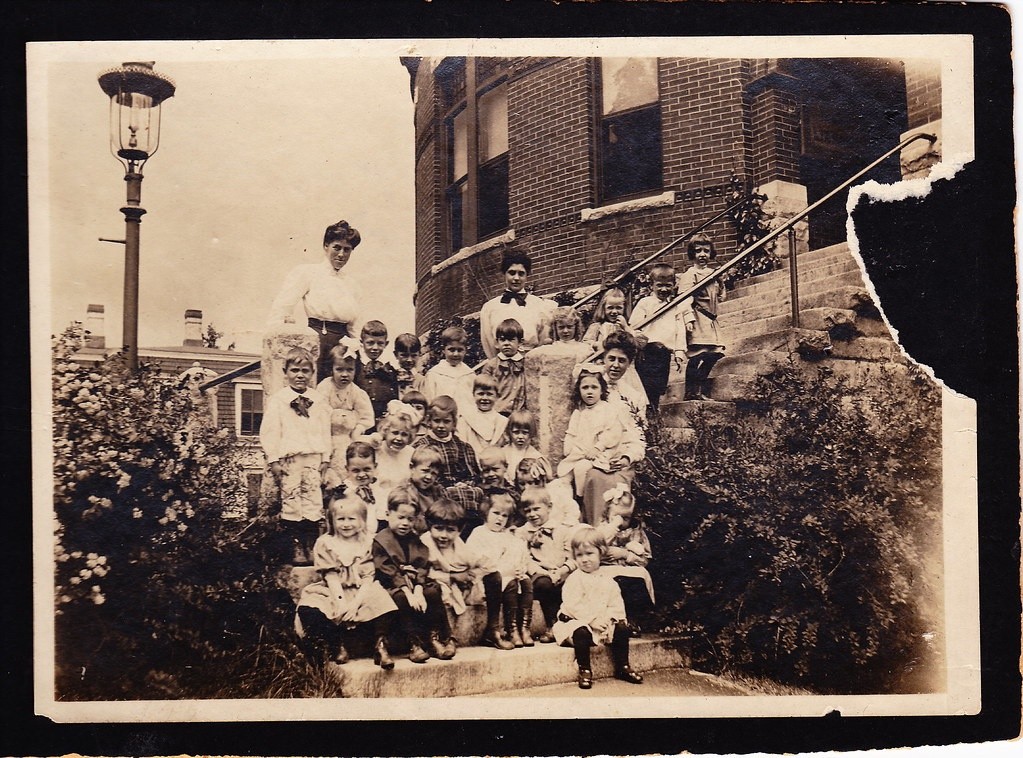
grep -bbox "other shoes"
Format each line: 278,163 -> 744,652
373,642 -> 395,669
539,627 -> 556,642
334,645 -> 350,664
481,628 -> 513,650
578,665 -> 593,688
613,665 -> 643,684
407,640 -> 431,662
626,617 -> 642,638
428,637 -> 446,659
442,639 -> 456,658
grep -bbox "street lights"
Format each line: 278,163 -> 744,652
98,60 -> 177,371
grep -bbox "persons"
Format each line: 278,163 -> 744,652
258,221 -> 728,688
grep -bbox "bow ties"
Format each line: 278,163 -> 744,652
500,291 -> 528,306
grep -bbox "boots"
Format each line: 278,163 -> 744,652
505,607 -> 523,648
518,606 -> 534,647
